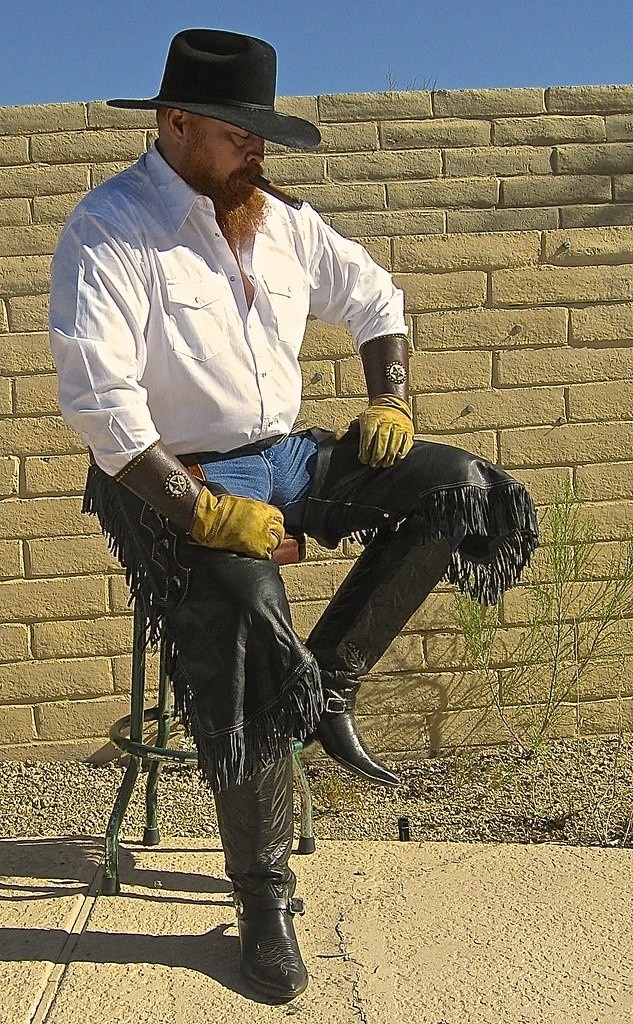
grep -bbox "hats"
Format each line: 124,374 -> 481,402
106,29 -> 323,150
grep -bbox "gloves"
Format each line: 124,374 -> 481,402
357,397 -> 417,470
189,485 -> 285,559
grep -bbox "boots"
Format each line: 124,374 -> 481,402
210,752 -> 308,998
304,511 -> 454,788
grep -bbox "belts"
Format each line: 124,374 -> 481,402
172,434 -> 284,467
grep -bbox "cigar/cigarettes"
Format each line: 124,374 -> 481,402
248,174 -> 304,210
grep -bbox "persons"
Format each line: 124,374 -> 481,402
50,28 -> 477,1003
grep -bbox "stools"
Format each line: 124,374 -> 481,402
100,527 -> 314,897
